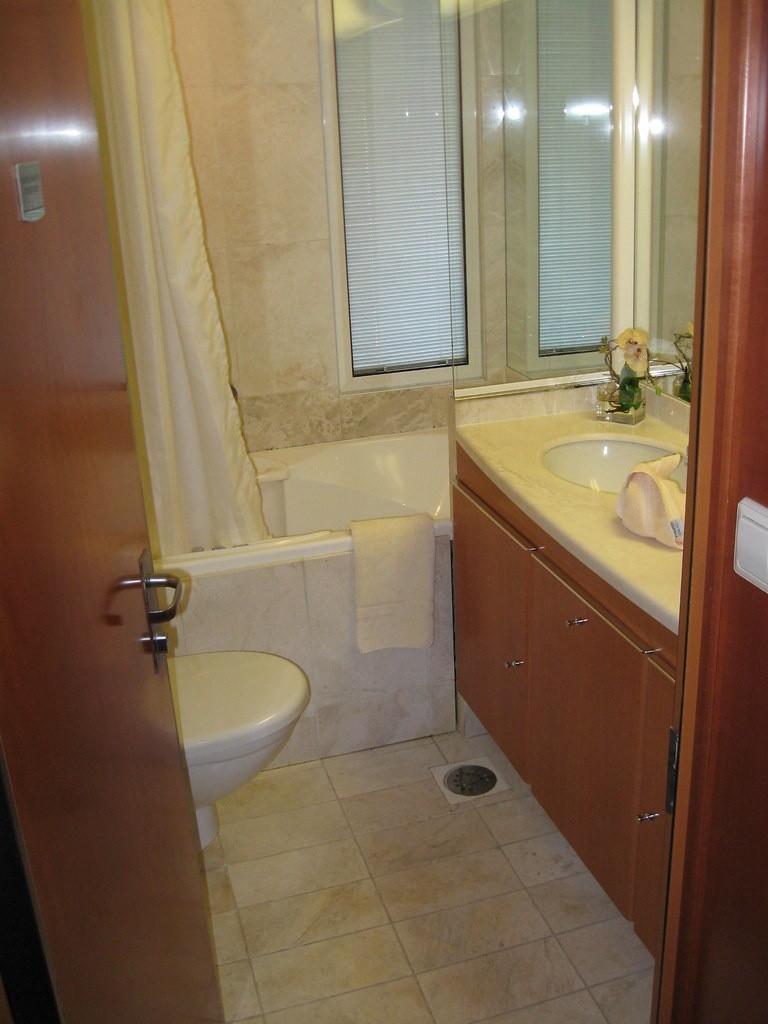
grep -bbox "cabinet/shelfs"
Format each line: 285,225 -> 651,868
634,656 -> 677,961
451,477 -> 539,784
529,548 -> 655,920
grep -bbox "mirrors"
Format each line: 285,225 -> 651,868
631,1 -> 705,404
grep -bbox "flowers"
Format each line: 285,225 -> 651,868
598,327 -> 662,414
648,322 -> 694,402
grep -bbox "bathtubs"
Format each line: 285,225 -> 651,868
163,426 -> 456,775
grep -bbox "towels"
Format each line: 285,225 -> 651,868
349,514 -> 437,654
616,453 -> 686,551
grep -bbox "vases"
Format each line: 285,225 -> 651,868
672,370 -> 691,402
597,381 -> 646,426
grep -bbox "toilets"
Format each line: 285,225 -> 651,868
166,650 -> 311,851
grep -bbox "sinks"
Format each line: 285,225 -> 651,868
538,434 -> 687,495
